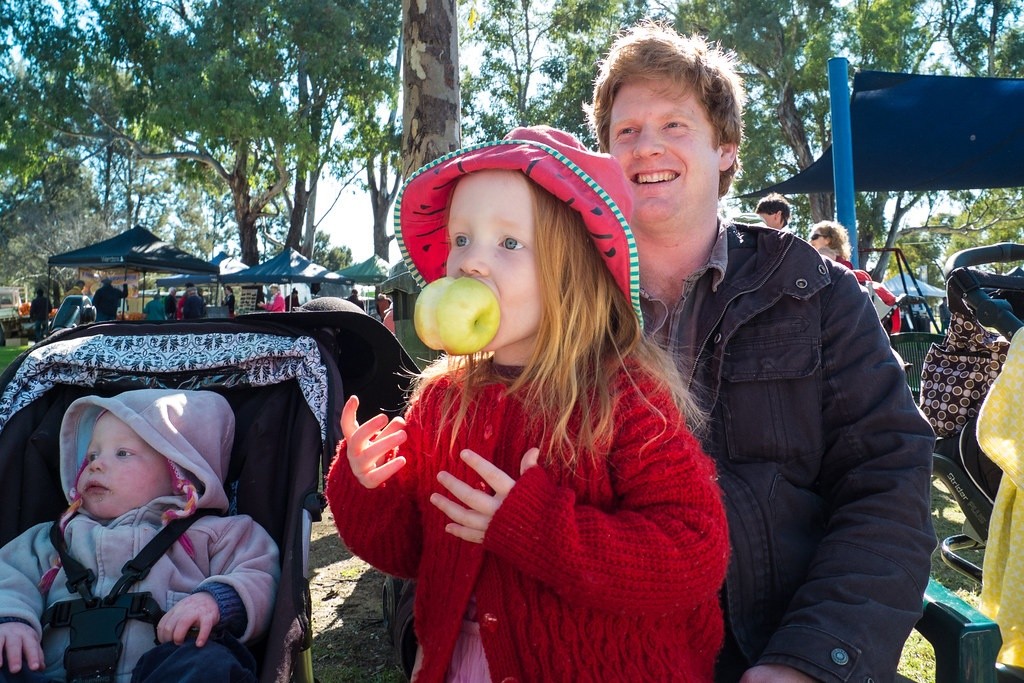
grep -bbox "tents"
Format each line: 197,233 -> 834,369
219,246 -> 354,311
45,224 -> 219,338
156,252 -> 251,307
319,254 -> 393,315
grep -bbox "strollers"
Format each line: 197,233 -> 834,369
0,320 -> 347,683
920,244 -> 1024,588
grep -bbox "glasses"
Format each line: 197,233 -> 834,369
783,216 -> 788,228
811,233 -> 829,240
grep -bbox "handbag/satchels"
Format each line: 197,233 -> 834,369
920,311 -> 1010,438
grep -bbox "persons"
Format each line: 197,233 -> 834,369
322,125 -> 731,683
590,28 -> 937,683
221,285 -> 235,318
0,389 -> 280,683
344,289 -> 364,311
733,192 -> 853,271
143,282 -> 208,319
258,284 -> 299,312
376,293 -> 395,334
30,277 -> 128,343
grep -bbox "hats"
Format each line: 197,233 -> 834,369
101,278 -> 112,283
394,125 -> 645,343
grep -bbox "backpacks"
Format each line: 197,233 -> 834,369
845,267 -> 901,337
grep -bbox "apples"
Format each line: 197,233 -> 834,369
414,276 -> 500,355
